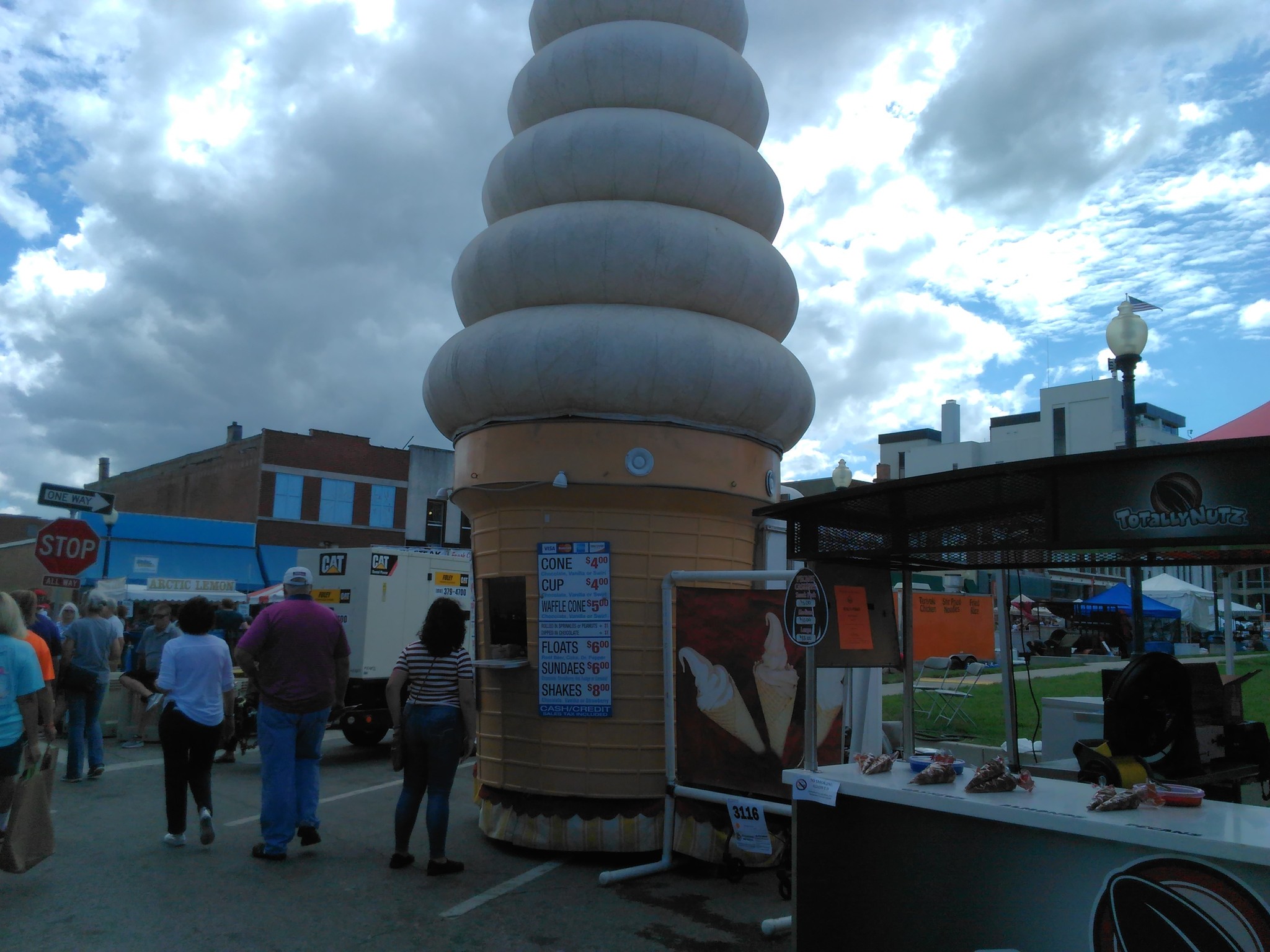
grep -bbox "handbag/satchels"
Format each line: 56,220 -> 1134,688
59,661 -> 97,695
0,758 -> 56,874
391,736 -> 404,770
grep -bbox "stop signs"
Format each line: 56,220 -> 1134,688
33,517 -> 101,578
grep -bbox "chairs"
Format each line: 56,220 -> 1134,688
912,656 -> 952,719
935,662 -> 985,728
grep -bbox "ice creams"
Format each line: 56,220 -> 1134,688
753,611 -> 799,758
423,1 -> 815,857
679,647 -> 766,754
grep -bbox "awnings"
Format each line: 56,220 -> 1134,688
257,545 -> 315,587
87,580 -> 246,610
64,538 -> 110,587
108,540 -> 266,591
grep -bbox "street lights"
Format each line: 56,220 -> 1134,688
1105,298 -> 1152,661
100,508 -> 119,577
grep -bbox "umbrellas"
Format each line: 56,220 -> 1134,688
246,582 -> 285,607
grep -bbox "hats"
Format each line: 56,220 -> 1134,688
283,567 -> 313,585
34,590 -> 50,608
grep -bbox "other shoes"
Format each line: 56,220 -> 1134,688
427,859 -> 464,876
251,844 -> 287,860
301,827 -> 321,845
87,764 -> 105,777
388,854 -> 415,868
62,773 -> 82,782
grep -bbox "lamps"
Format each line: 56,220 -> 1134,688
552,470 -> 568,488
434,487 -> 453,501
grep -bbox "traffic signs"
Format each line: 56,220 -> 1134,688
38,482 -> 115,515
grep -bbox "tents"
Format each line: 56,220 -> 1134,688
994,595 -> 1059,627
1190,599 -> 1265,643
1072,583 -> 1183,644
1128,574 -> 1216,644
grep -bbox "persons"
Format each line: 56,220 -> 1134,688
494,643 -> 528,659
118,603 -> 184,748
387,596 -> 476,877
1231,621 -> 1268,653
58,591 -> 122,782
1036,627 -> 1129,660
125,600 -> 186,634
8,589 -> 59,743
33,589 -> 129,736
208,599 -> 262,660
234,567 -> 352,861
152,594 -> 234,846
1117,609 -> 1132,641
1010,618 -> 1028,631
0,591 -> 45,846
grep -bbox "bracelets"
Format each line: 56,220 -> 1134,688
46,722 -> 54,729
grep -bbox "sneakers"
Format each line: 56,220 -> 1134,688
164,833 -> 184,845
122,739 -> 144,748
200,809 -> 214,845
145,693 -> 164,714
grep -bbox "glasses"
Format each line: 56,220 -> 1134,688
152,614 -> 167,619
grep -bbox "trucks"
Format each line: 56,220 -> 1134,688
296,547 -> 475,745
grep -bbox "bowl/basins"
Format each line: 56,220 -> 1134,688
1132,784 -> 1204,807
908,755 -> 966,775
